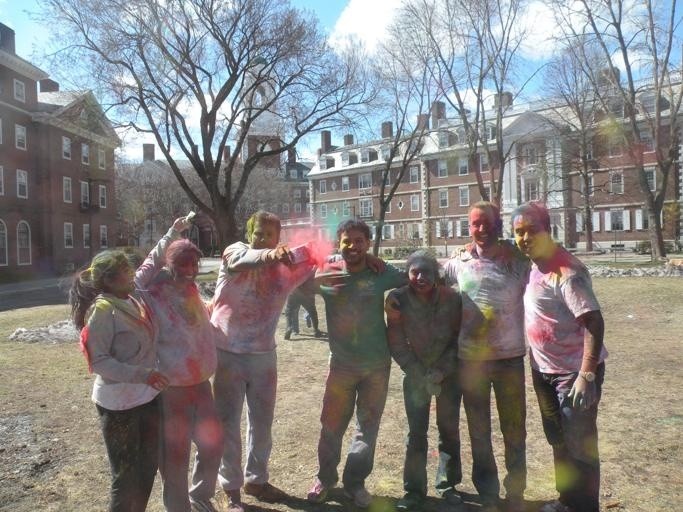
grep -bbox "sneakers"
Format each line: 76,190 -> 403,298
307,482 -> 329,504
436,486 -> 462,504
190,499 -> 217,512
396,496 -> 421,510
540,500 -> 566,512
343,487 -> 368,508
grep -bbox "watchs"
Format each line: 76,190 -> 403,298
578,371 -> 598,383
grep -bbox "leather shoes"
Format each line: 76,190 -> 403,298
245,482 -> 281,500
228,503 -> 245,512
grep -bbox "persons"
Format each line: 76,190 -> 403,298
138,239 -> 224,512
214,210 -> 387,512
384,201 -> 531,512
69,217 -> 193,512
387,249 -> 463,512
283,274 -> 327,340
296,218 -> 410,508
510,201 -> 608,512
292,306 -> 311,335
